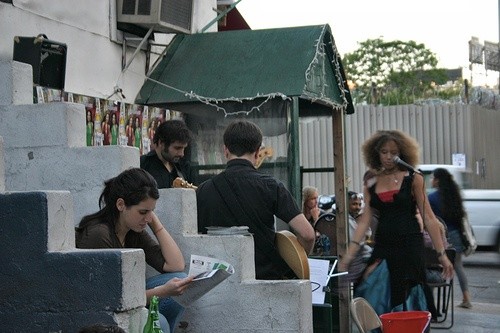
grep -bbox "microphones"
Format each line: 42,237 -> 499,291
394,156 -> 422,174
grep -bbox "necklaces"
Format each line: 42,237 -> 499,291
382,169 -> 399,184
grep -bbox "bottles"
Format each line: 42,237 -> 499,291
144,296 -> 163,333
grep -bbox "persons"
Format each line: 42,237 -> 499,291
139,118 -> 201,190
300,167 -> 380,299
87,110 -> 160,156
420,167 -> 477,310
192,119 -> 316,282
336,129 -> 455,333
74,167 -> 195,333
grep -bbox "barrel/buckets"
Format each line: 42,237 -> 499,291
380,310 -> 432,333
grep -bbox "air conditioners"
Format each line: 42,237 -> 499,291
117,0 -> 194,34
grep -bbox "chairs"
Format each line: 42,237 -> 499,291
429,247 -> 457,330
350,297 -> 382,333
314,213 -> 337,254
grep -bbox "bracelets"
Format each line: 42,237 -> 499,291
155,226 -> 164,233
437,250 -> 445,257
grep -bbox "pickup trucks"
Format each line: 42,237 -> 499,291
416,164 -> 500,253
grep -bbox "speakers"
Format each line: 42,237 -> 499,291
32,37 -> 68,91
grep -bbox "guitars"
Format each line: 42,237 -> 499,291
172,176 -> 311,280
255,147 -> 273,168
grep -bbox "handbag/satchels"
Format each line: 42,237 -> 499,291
461,209 -> 477,256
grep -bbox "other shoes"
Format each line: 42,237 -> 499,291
457,301 -> 473,309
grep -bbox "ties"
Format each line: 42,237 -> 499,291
169,162 -> 178,187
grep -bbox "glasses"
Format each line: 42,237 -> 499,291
350,194 -> 362,199
